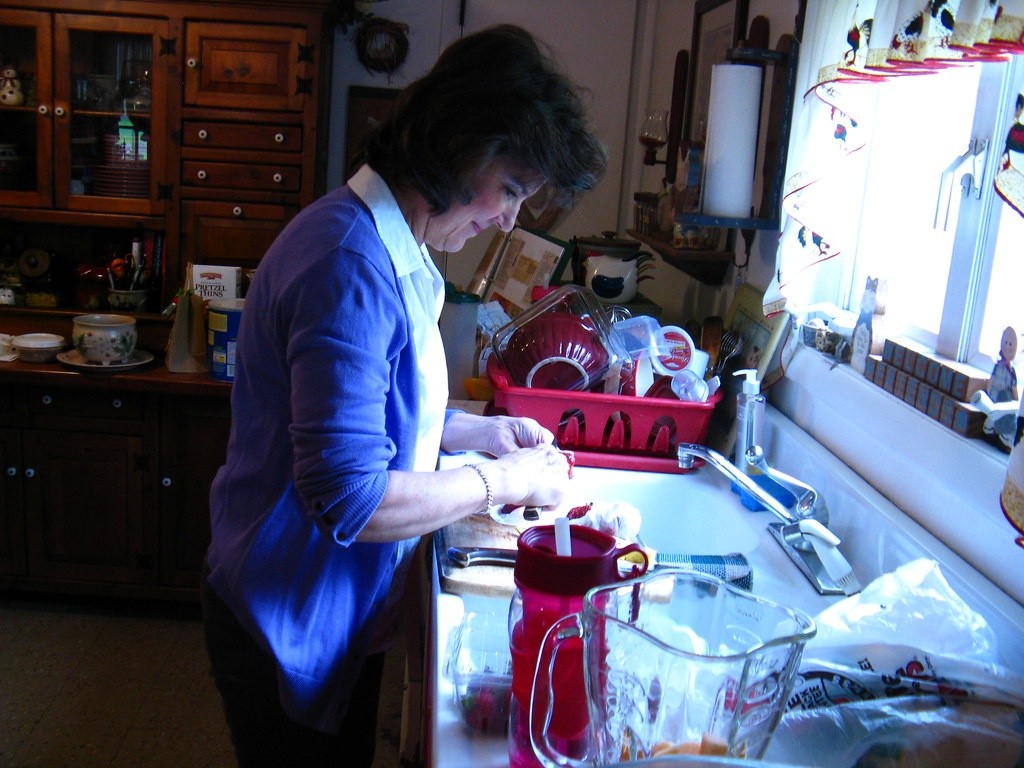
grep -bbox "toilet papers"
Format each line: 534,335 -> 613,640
695,63 -> 764,221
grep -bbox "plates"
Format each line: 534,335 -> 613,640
93,133 -> 151,198
56,348 -> 154,371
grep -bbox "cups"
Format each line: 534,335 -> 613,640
507,525 -> 648,768
72,313 -> 137,364
72,138 -> 95,164
639,109 -> 669,151
530,569 -> 817,768
74,79 -> 87,105
109,286 -> 148,314
440,290 -> 481,401
673,221 -> 704,249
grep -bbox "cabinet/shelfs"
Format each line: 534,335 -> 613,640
0,354 -> 236,602
0,0 -> 335,327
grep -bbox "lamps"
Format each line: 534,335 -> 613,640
639,100 -> 672,166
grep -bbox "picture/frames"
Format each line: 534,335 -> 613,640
682,0 -> 749,151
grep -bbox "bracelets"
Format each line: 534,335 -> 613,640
463,465 -> 493,516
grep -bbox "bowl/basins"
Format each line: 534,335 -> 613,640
14,334 -> 64,363
493,284 -> 721,400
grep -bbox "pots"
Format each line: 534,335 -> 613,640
574,231 -> 640,290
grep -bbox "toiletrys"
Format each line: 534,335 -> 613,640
732,366 -> 768,493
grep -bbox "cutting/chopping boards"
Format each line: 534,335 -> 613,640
438,455 -> 633,596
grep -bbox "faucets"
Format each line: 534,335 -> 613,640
677,440 -> 808,529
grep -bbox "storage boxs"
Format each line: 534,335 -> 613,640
480,344 -> 726,475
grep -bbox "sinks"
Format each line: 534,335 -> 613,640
431,449 -> 774,577
429,570 -> 847,767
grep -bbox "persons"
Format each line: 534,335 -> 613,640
201,21 -> 607,768
